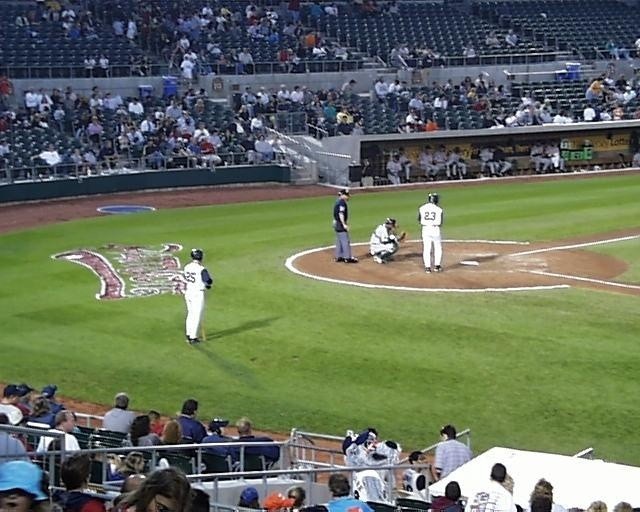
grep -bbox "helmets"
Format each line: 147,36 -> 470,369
427,192 -> 439,203
288,486 -> 305,505
191,248 -> 203,261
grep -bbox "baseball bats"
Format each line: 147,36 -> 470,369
201,320 -> 206,341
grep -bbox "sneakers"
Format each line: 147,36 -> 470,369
337,255 -> 444,274
186,336 -> 200,344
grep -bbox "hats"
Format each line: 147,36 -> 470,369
4,382 -> 35,399
386,218 -> 395,225
41,385 -> 57,399
262,493 -> 296,508
0,460 -> 48,502
208,418 -> 229,435
240,488 -> 258,504
338,189 -> 350,196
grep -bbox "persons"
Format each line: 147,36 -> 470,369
3,2 -> 639,185
331,188 -> 360,264
3,383 -> 636,512
368,217 -> 406,263
418,191 -> 444,274
181,247 -> 215,345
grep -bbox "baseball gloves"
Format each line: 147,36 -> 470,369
397,232 -> 406,241
